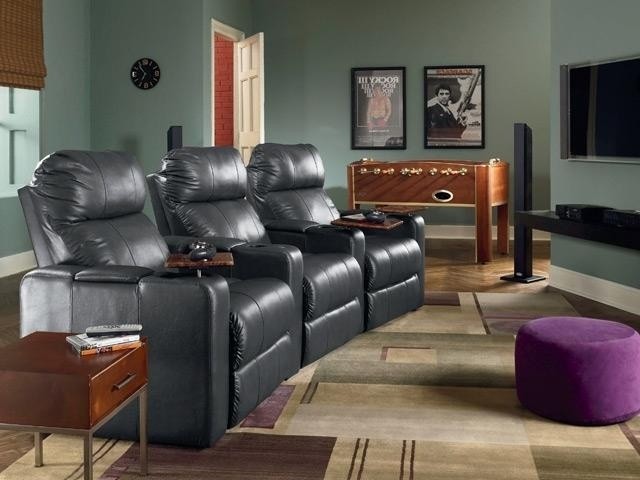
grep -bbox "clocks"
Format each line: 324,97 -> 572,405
127,58 -> 163,90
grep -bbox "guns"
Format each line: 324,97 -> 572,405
455,70 -> 480,129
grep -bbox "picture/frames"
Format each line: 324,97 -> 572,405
349,64 -> 486,151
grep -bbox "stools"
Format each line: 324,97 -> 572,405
516,314 -> 640,425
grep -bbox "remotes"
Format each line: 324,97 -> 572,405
86,323 -> 143,338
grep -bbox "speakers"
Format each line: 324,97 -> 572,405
499,122 -> 545,282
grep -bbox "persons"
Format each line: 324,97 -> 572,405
426,83 -> 469,138
365,83 -> 393,130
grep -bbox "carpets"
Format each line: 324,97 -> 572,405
0,290 -> 640,480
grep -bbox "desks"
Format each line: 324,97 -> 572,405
0,331 -> 150,479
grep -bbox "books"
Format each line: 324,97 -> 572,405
63,331 -> 144,356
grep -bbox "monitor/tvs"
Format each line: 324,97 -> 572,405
559,55 -> 640,164
